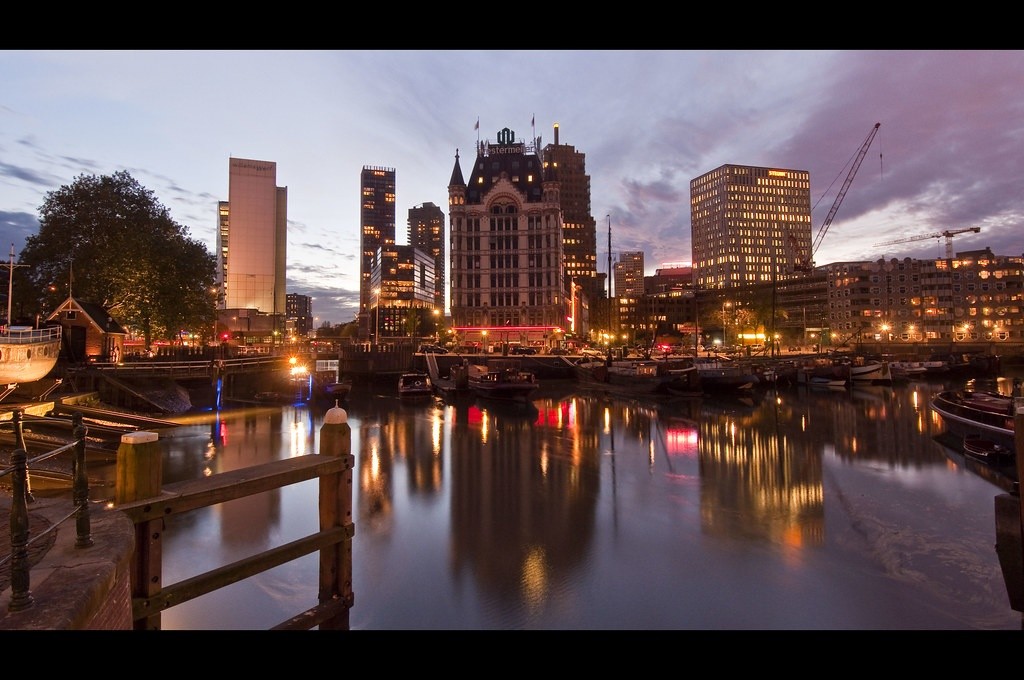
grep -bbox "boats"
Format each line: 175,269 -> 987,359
558,340 -> 666,394
928,375 -> 1024,465
326,382 -> 351,398
450,344 -> 541,401
667,351 -> 997,388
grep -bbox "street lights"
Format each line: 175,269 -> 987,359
481,330 -> 487,365
373,287 -> 379,346
233,316 -> 236,331
605,214 -> 613,367
722,302 -> 731,349
567,276 -> 578,331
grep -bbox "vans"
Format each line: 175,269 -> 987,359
418,343 -> 448,355
509,345 -> 537,355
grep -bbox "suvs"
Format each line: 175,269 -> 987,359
577,347 -> 603,356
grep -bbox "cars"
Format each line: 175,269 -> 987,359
657,344 -> 767,352
788,345 -> 801,352
549,347 -> 571,356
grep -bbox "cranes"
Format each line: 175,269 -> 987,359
872,226 -> 980,259
811,122 -> 883,265
